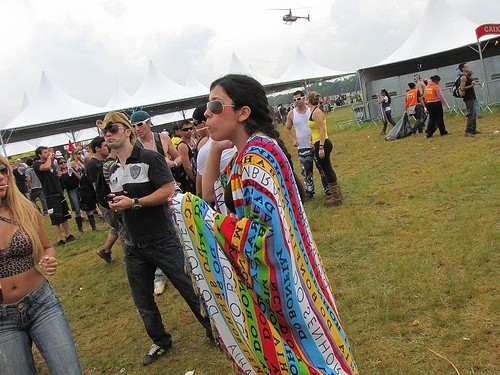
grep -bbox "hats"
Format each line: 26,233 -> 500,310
96,112 -> 136,145
17,158 -> 21,162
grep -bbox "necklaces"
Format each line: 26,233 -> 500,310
296,107 -> 306,116
137,132 -> 155,151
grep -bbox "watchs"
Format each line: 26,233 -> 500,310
133,198 -> 142,209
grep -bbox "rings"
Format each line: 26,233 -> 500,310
115,210 -> 117,212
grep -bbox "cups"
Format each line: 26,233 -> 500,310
109,179 -> 125,197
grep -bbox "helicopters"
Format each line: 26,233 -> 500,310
269,6 -> 310,25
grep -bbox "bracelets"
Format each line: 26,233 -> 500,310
173,160 -> 177,168
319,145 -> 324,149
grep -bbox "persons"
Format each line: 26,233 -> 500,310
0,156 -> 83,375
405,74 -> 433,134
451,62 -> 482,137
306,91 -> 344,205
88,110 -> 184,296
377,88 -> 397,135
269,90 -> 363,125
286,89 -> 331,199
172,75 -> 359,375
96,112 -> 220,366
423,74 -> 451,138
0,103 -> 237,246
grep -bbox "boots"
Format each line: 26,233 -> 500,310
76,216 -> 83,234
324,181 -> 344,206
88,214 -> 96,232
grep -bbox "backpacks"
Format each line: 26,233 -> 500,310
452,74 -> 465,98
76,159 -> 102,212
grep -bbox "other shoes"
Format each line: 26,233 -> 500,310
304,193 -> 313,202
65,234 -> 75,243
57,239 -> 68,247
96,249 -> 112,263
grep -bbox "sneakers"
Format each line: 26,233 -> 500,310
143,334 -> 172,366
154,278 -> 167,295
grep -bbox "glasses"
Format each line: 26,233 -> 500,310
206,100 -> 235,115
193,121 -> 200,127
59,161 -> 67,165
0,165 -> 8,175
293,96 -> 304,101
100,124 -> 126,137
131,118 -> 149,127
181,127 -> 192,131
